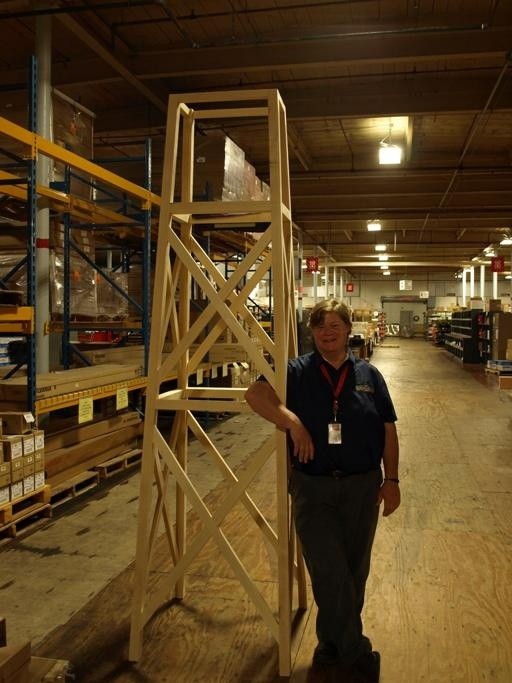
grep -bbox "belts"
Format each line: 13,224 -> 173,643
328,469 -> 346,481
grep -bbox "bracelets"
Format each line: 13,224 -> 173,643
384,479 -> 399,482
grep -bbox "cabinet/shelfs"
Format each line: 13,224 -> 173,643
444,308 -> 492,363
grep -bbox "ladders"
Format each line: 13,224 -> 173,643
130,89 -> 306,676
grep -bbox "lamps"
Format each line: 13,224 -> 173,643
379,123 -> 402,165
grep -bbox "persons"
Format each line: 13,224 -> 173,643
245,298 -> 402,683
